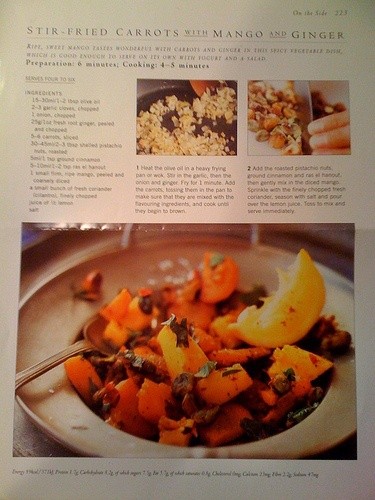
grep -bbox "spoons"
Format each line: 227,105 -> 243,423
14,315 -> 120,394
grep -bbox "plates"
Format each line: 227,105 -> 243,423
14,224 -> 357,458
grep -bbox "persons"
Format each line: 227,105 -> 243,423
306,90 -> 350,155
